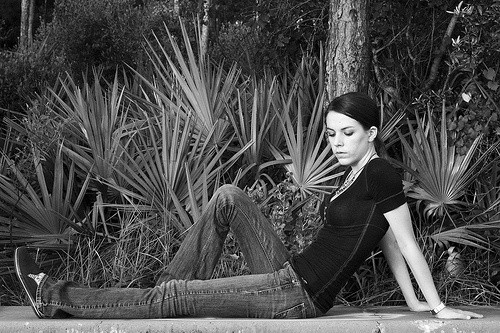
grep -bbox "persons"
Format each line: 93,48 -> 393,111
8,92 -> 484,325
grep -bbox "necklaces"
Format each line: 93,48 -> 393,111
329,152 -> 378,197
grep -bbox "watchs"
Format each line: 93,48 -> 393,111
429,302 -> 447,315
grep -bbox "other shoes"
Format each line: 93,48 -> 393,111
15,246 -> 49,320
